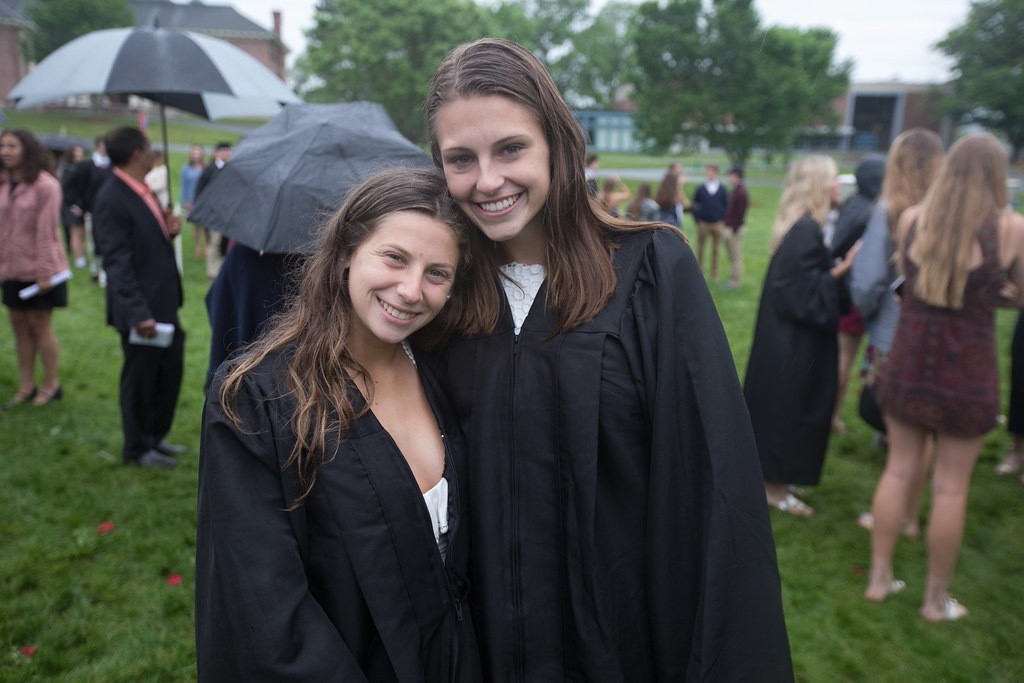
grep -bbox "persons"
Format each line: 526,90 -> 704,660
91,124 -> 192,473
181,143 -> 235,264
995,308 -> 1024,481
143,148 -> 169,209
833,157 -> 886,430
742,154 -> 864,518
1,128 -> 68,406
424,38 -> 801,683
204,238 -> 308,395
585,154 -> 689,229
721,164 -> 748,286
848,127 -> 949,538
48,137 -> 112,283
690,164 -> 727,280
863,133 -> 1024,622
195,168 -> 513,683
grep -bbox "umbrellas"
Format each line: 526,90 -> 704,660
187,100 -> 437,256
4,17 -> 306,206
33,132 -> 92,155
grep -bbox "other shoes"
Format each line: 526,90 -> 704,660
31,385 -> 61,406
766,494 -> 815,517
5,386 -> 37,407
154,443 -> 186,455
125,450 -> 177,469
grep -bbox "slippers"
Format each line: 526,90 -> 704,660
864,579 -> 905,599
919,598 -> 969,622
